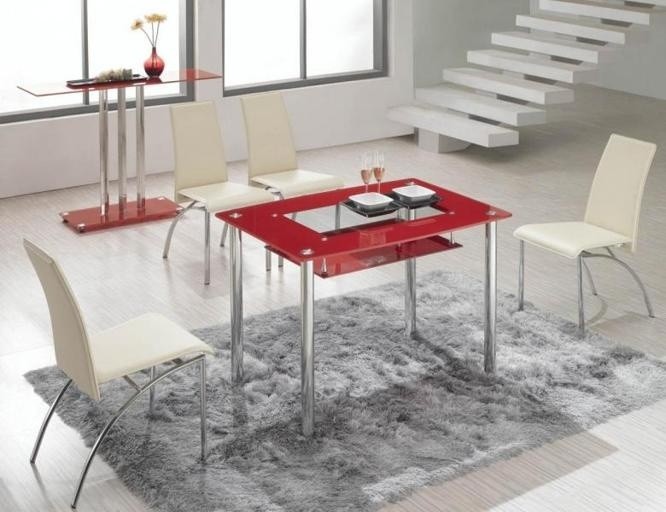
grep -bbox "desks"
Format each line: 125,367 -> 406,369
214,174 -> 513,439
17,66 -> 224,232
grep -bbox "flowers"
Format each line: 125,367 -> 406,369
129,14 -> 167,45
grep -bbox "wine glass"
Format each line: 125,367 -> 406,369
360,151 -> 385,193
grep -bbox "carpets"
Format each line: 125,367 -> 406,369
21,268 -> 665,512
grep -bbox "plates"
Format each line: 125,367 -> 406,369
339,184 -> 442,217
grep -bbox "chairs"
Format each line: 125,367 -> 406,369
514,134 -> 656,333
164,91 -> 347,285
22,237 -> 207,509
